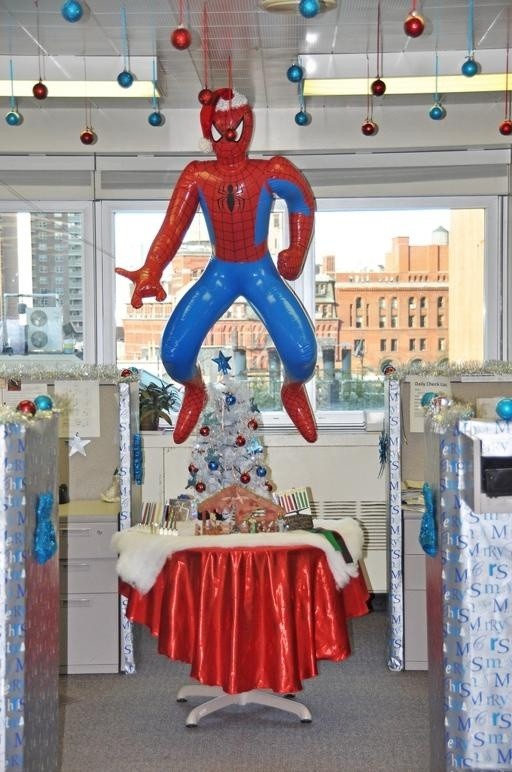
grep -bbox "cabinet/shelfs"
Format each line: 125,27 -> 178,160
59,523 -> 119,675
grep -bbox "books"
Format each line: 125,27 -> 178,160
401,478 -> 426,511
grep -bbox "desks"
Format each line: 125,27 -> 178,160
132,517 -> 365,726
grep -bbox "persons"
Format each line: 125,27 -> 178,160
114,87 -> 318,444
